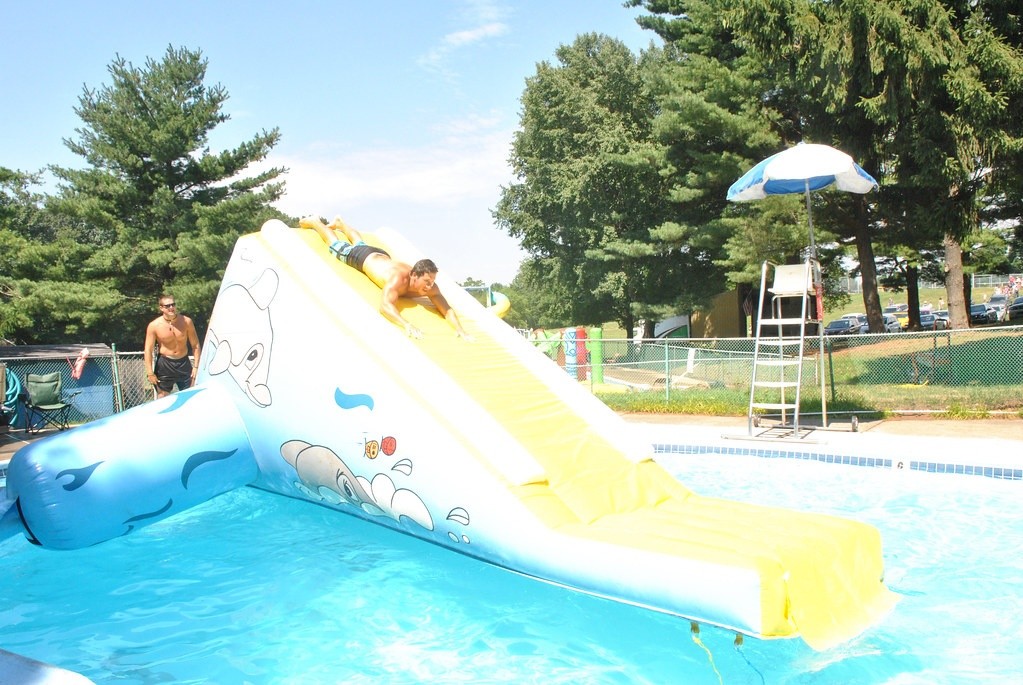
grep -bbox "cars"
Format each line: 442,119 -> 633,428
822,302 -> 949,341
1009,296 -> 1023,323
988,295 -> 1007,313
970,303 -> 998,324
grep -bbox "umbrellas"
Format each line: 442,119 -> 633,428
727,141 -> 879,284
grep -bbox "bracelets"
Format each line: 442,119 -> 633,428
194,366 -> 198,369
146,374 -> 155,377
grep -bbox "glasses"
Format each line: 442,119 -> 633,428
162,303 -> 175,309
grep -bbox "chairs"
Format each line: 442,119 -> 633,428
912,316 -> 953,386
18,371 -> 71,435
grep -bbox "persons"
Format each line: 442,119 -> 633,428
299,216 -> 478,343
144,294 -> 202,400
887,275 -> 1023,310
529,325 -> 561,360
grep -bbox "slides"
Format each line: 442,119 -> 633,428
260,213 -> 888,645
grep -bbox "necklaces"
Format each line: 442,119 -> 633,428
163,313 -> 177,331
